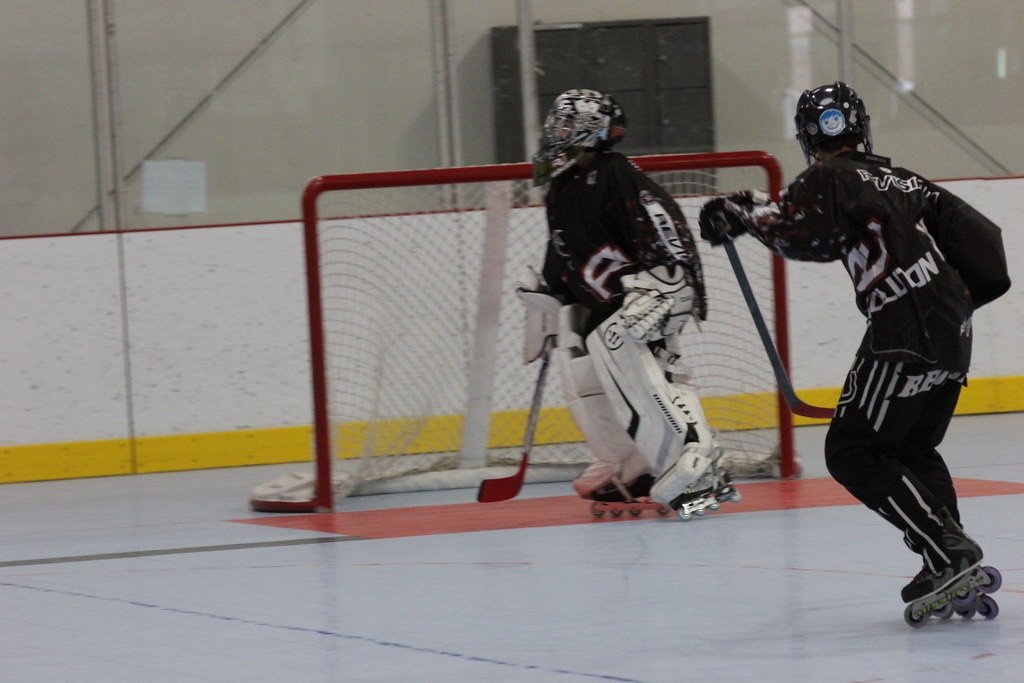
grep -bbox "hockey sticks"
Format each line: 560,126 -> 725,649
725,236 -> 836,419
478,335 -> 553,503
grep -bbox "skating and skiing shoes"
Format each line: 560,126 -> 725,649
590,472 -> 671,517
672,462 -> 741,521
901,508 -> 1002,628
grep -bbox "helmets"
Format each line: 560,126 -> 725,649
533,88 -> 628,188
794,81 -> 873,166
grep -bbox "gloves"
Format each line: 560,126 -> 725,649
699,190 -> 770,245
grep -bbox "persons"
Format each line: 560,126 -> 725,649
699,80 -> 1012,603
514,88 -> 730,507
